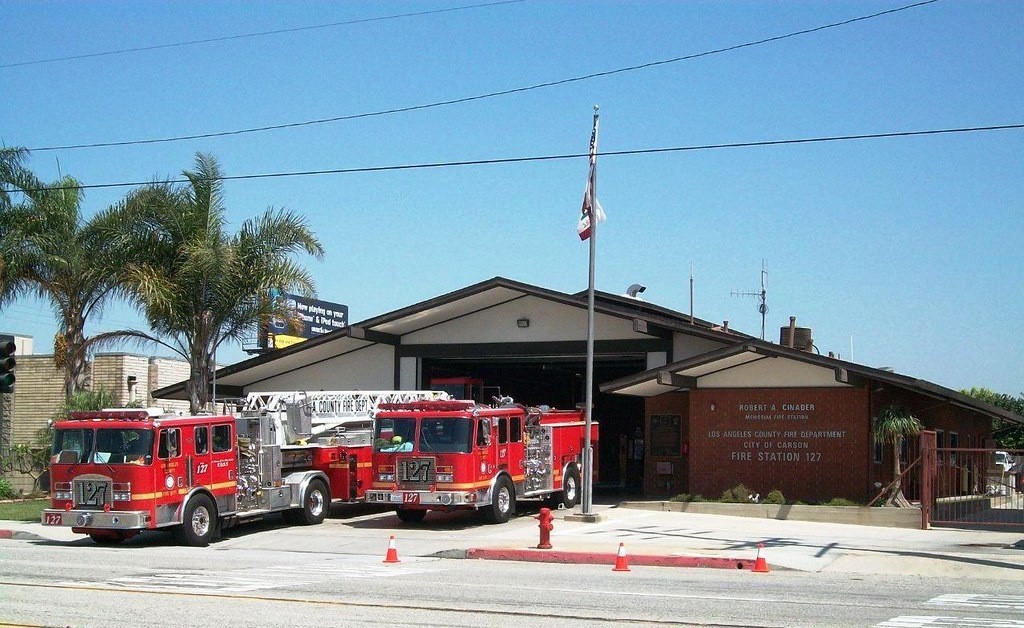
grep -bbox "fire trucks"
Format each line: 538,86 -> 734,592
365,394 -> 599,523
40,391 -> 451,547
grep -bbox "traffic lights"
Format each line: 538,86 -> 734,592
0,334 -> 16,394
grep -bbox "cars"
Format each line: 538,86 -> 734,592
1007,462 -> 1024,494
995,451 -> 1016,472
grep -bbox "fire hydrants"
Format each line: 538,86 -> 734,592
534,509 -> 554,550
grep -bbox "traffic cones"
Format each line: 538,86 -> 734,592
752,543 -> 769,572
611,542 -> 631,571
383,535 -> 402,562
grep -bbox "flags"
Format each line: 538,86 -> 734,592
578,111 -> 606,241
